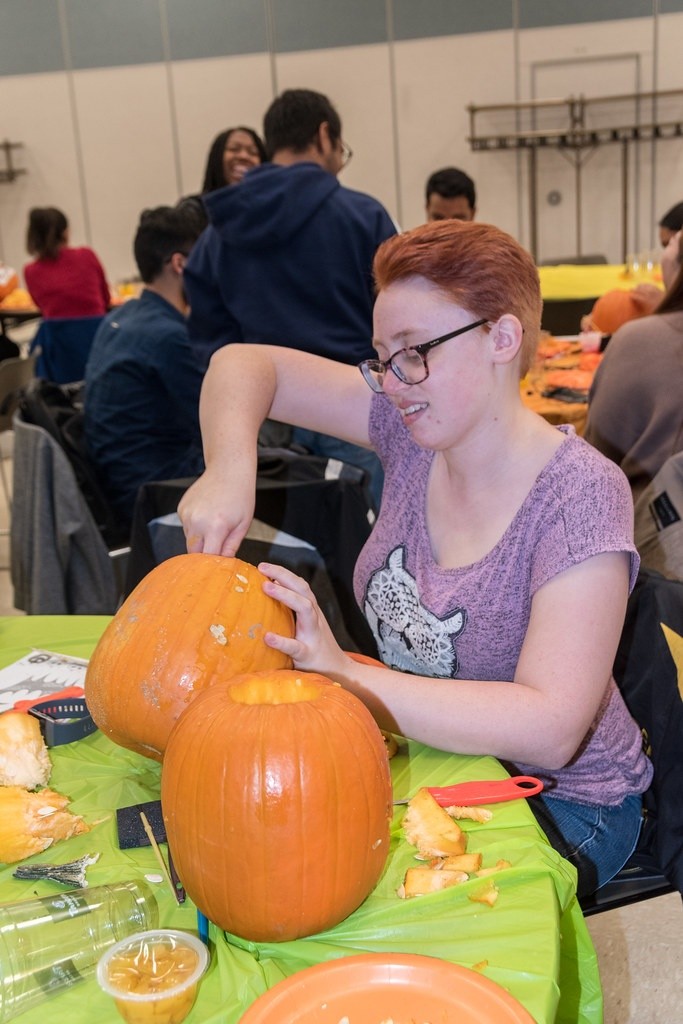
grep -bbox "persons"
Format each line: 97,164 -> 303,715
583,202 -> 682,496
188,125 -> 266,223
82,202 -> 193,539
425,168 -> 476,222
22,207 -> 111,393
177,221 -> 655,899
180,89 -> 395,513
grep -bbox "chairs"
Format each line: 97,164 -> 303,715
31,317 -> 107,383
577,570 -> 683,918
0,346 -> 380,661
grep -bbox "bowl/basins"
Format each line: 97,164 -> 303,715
96,928 -> 209,1024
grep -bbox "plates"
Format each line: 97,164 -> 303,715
235,952 -> 536,1024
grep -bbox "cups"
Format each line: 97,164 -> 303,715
0,879 -> 159,1024
598,332 -> 611,351
579,331 -> 601,354
627,251 -> 658,280
538,329 -> 550,373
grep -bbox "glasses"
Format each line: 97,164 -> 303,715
358,320 -> 487,395
337,137 -> 353,168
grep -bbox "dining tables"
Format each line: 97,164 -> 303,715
517,335 -> 614,440
0,615 -> 603,1024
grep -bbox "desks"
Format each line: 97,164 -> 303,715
534,260 -> 667,305
2,276 -> 151,314
